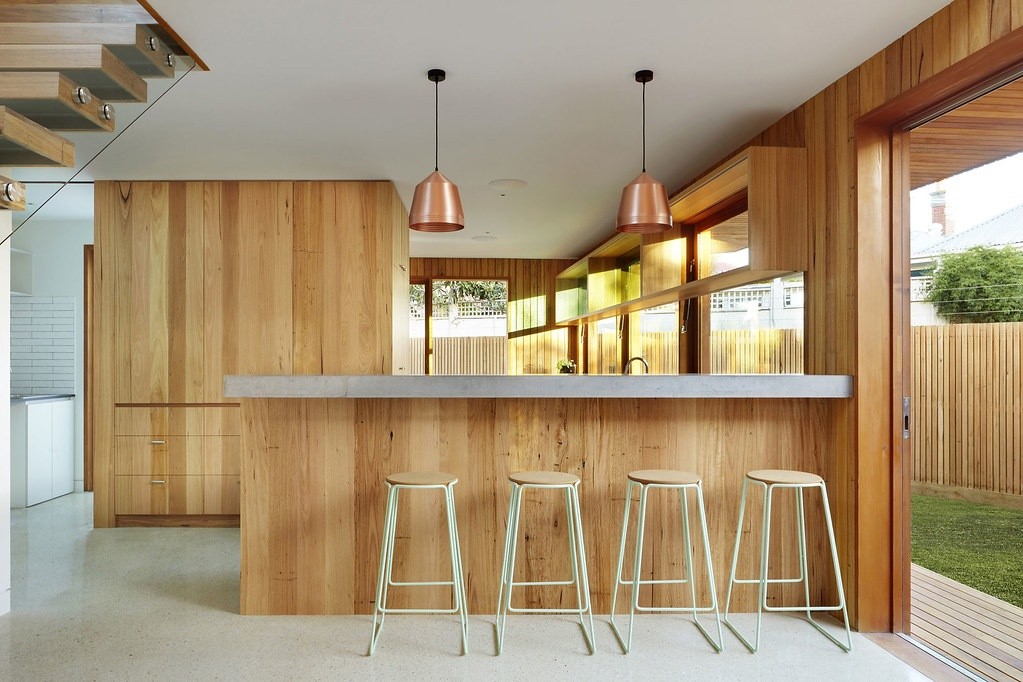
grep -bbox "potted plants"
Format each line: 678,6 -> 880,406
557,359 -> 577,374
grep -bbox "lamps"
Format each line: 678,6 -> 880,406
617,69 -> 671,233
407,69 -> 465,233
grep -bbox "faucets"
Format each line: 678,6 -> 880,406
624,357 -> 649,374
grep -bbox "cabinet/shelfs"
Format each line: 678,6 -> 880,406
9,394 -> 75,509
113,403 -> 242,527
555,146 -> 808,325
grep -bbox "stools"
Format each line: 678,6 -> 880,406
611,470 -> 724,654
725,469 -> 853,654
495,471 -> 597,655
368,471 -> 469,656
